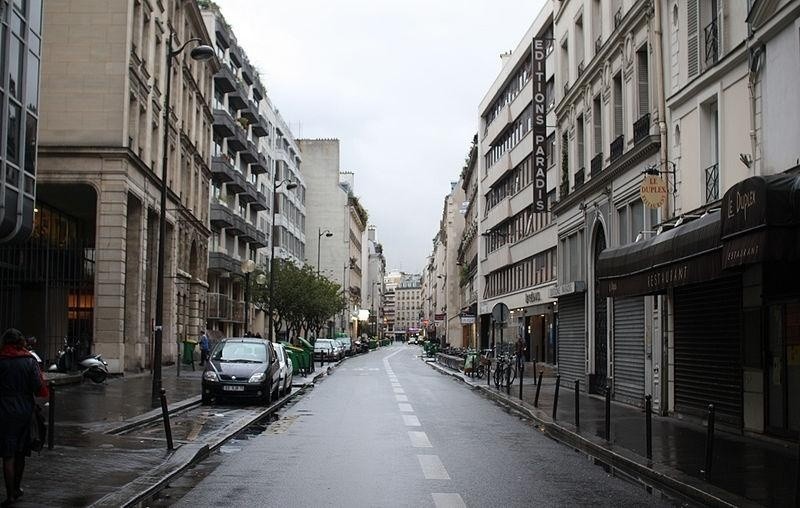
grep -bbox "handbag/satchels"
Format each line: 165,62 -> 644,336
22,403 -> 45,453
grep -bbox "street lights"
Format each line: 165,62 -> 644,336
269,173 -> 298,342
343,262 -> 356,334
317,226 -> 333,278
151,23 -> 214,408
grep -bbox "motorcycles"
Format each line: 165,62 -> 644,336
47,334 -> 109,383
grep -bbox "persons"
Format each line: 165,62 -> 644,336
0,328 -> 49,506
197,330 -> 208,366
515,333 -> 527,372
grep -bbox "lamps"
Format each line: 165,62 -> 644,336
481,228 -> 506,238
456,261 -> 467,265
437,273 -> 447,280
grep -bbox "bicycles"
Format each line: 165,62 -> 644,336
434,346 -> 518,386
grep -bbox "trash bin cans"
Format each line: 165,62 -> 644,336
369,338 -> 390,348
276,337 -> 315,376
424,341 -> 430,351
183,339 -> 198,364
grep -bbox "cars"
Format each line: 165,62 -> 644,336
408,337 -> 418,344
202,335 -> 281,406
271,342 -> 293,396
314,337 -> 352,362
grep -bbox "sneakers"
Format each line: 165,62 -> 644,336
5,487 -> 24,504
198,363 -> 205,367
